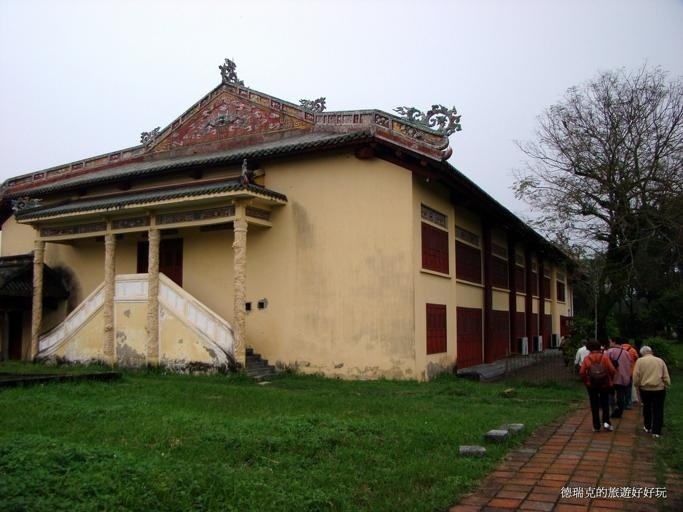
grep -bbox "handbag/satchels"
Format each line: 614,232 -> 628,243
612,360 -> 619,369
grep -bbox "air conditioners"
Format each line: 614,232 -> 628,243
517,333 -> 561,356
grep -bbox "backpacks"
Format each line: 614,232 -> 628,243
588,353 -> 608,385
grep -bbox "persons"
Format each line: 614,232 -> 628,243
599,342 -> 606,354
632,345 -> 671,438
566,334 -> 574,345
604,336 -> 632,418
574,339 -> 591,368
579,340 -> 618,432
559,335 -> 570,367
620,338 -> 640,406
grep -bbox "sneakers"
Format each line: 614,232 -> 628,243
603,422 -> 614,431
643,425 -> 659,439
611,405 -> 619,418
591,424 -> 600,432
